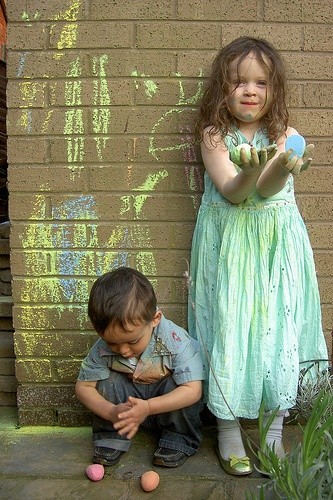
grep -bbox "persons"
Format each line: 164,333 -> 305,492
188,36 -> 328,475
75,266 -> 207,467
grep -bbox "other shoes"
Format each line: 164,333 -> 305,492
277,443 -> 285,460
93,446 -> 124,465
153,447 -> 188,467
216,442 -> 252,474
